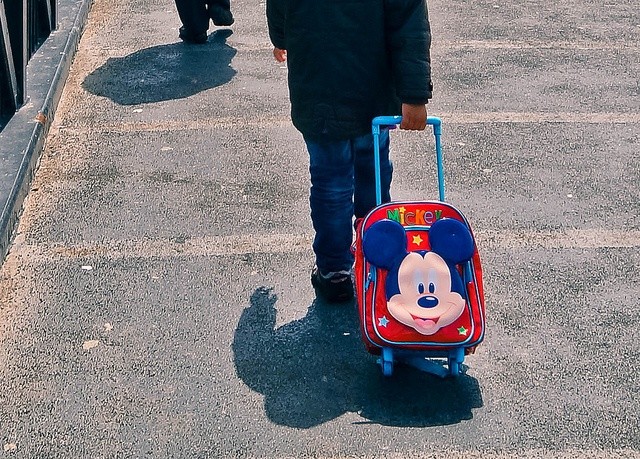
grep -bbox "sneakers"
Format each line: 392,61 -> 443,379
179,25 -> 208,43
310,261 -> 355,301
208,4 -> 234,26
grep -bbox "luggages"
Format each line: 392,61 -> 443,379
349,116 -> 486,377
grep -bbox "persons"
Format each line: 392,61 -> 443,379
266,0 -> 433,297
174,0 -> 235,43
